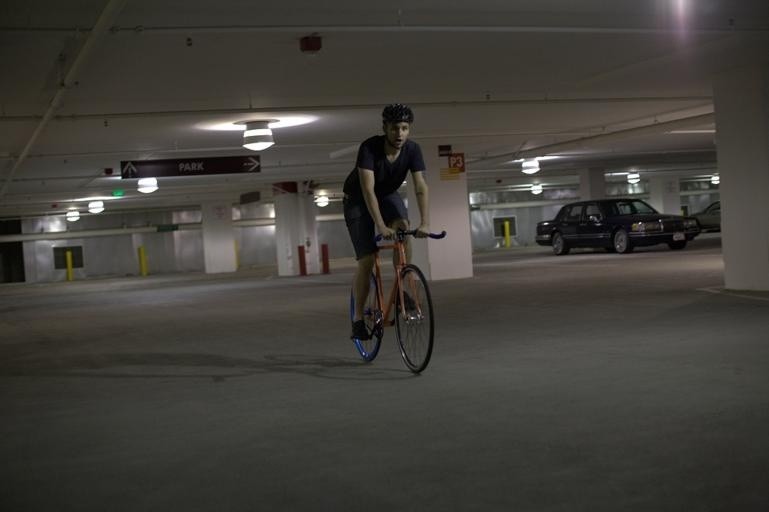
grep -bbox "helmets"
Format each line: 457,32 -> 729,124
382,104 -> 414,122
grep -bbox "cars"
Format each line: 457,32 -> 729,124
535,198 -> 701,255
691,202 -> 721,231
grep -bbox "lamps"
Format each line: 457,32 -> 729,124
711,177 -> 720,185
627,174 -> 640,183
316,197 -> 329,208
531,185 -> 542,195
242,122 -> 276,153
66,211 -> 80,222
137,177 -> 159,194
88,201 -> 104,214
521,159 -> 540,175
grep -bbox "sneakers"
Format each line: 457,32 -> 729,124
351,319 -> 368,340
393,292 -> 416,310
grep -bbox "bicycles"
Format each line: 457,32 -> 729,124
350,230 -> 446,374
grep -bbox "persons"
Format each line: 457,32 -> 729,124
341,101 -> 431,342
